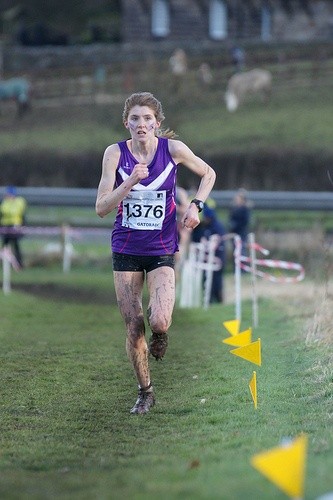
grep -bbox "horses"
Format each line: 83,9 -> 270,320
225,68 -> 273,112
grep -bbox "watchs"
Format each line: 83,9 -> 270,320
191,198 -> 205,213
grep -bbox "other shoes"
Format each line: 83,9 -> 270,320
149,331 -> 168,360
130,392 -> 155,415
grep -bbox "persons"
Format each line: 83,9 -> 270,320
95,91 -> 216,415
176,185 -> 224,304
1,185 -> 26,271
229,188 -> 250,274
